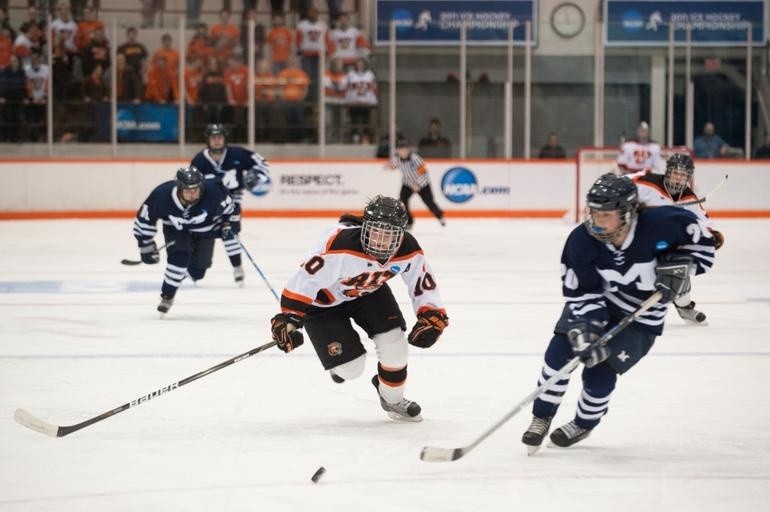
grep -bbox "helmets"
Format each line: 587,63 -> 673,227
664,153 -> 694,175
587,173 -> 637,210
176,166 -> 204,188
365,196 -> 408,232
205,123 -> 228,136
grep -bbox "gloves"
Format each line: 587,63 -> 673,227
271,313 -> 306,353
655,261 -> 691,304
566,318 -> 611,368
141,241 -> 159,264
408,310 -> 448,348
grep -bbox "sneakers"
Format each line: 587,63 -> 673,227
673,302 -> 705,322
522,416 -> 552,446
550,419 -> 590,447
372,375 -> 421,418
158,292 -> 173,312
234,266 -> 243,281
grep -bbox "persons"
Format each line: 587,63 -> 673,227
133,166 -> 235,313
190,122 -> 271,282
378,121 -> 419,156
345,56 -> 378,146
324,57 -> 348,143
389,139 -> 445,230
278,54 -> 310,142
617,122 -> 665,177
693,121 -> 730,158
271,196 -> 448,418
539,133 -> 565,158
522,172 -> 715,447
755,132 -> 769,157
417,118 -> 451,157
1,1 -> 111,143
116,8 -> 311,112
633,152 -> 724,323
296,7 -> 329,102
324,11 -> 369,58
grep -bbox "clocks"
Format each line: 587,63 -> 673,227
550,3 -> 585,38
